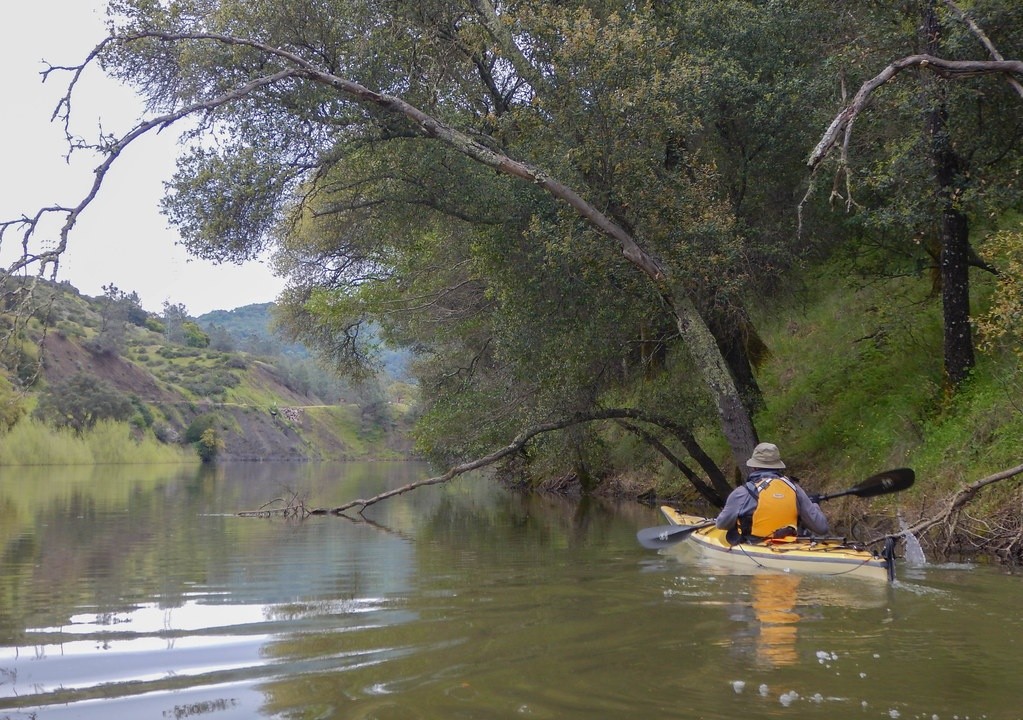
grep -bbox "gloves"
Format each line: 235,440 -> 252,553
808,494 -> 821,505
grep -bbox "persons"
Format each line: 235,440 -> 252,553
716,443 -> 829,545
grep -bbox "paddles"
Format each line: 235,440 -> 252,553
765,535 -> 847,544
635,466 -> 915,551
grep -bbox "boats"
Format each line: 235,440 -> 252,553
658,505 -> 891,583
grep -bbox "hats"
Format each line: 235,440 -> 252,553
746,442 -> 786,469
